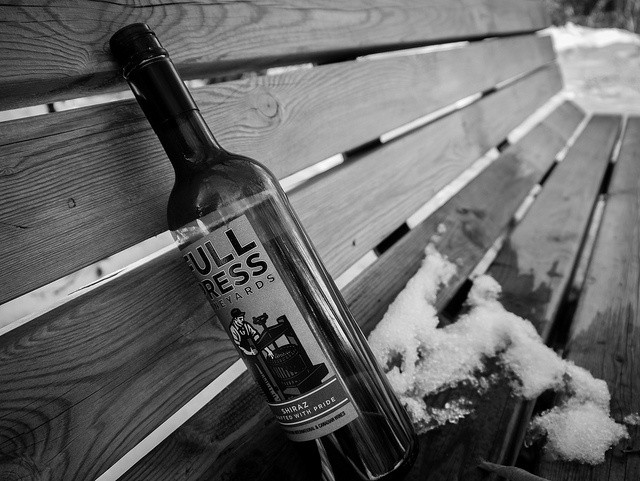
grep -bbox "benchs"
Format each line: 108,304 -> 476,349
0,1 -> 639,481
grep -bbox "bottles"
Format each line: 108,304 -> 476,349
103,23 -> 419,481
